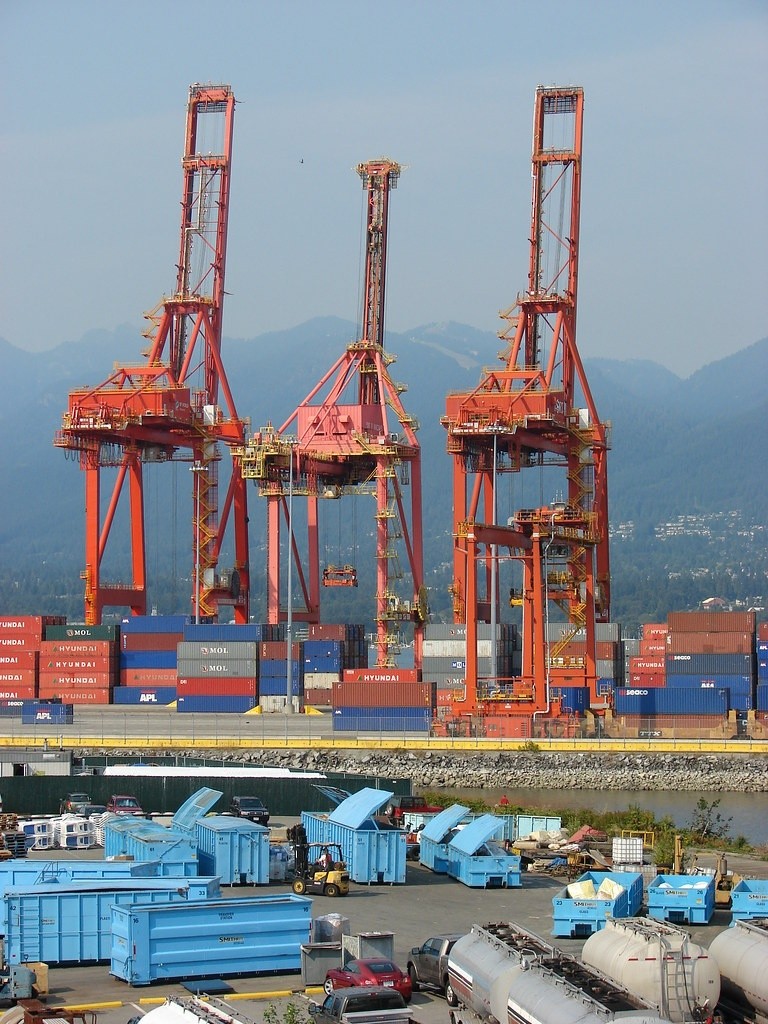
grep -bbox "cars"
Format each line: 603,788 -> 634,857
324,959 -> 413,1005
76,803 -> 107,818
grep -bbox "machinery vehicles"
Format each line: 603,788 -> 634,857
286,824 -> 350,897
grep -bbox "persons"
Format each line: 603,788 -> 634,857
307,847 -> 332,880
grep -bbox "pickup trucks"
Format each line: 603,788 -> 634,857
406,933 -> 460,1007
308,986 -> 421,1024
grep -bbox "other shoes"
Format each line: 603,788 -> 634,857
307,875 -> 313,880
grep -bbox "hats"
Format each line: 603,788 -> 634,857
321,848 -> 328,851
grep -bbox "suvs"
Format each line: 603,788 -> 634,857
385,795 -> 444,825
105,794 -> 143,816
230,796 -> 270,826
58,790 -> 92,815
